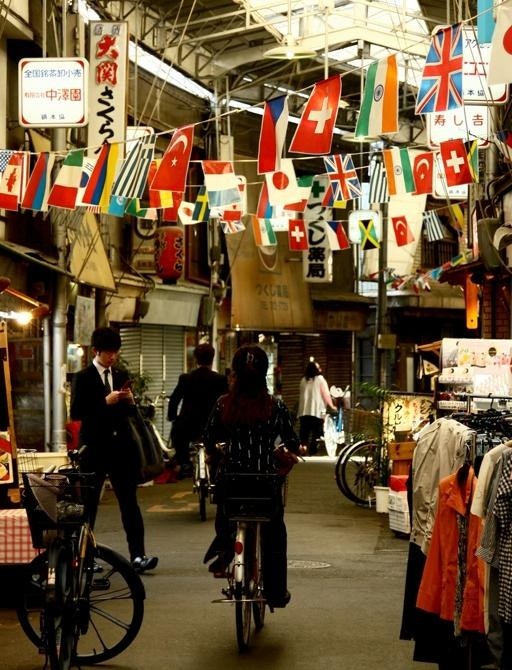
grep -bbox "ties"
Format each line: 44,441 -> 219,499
104,370 -> 113,393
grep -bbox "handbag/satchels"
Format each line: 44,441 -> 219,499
131,410 -> 163,483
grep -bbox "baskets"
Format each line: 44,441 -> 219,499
22,472 -> 96,532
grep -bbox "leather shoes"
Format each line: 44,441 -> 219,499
175,464 -> 194,481
133,556 -> 159,572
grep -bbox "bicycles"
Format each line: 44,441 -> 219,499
213,441 -> 304,652
16,444 -> 144,670
186,441 -> 215,520
336,434 -> 390,507
322,386 -> 350,458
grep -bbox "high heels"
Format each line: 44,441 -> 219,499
267,592 -> 289,613
207,552 -> 235,574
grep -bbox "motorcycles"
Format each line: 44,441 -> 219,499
135,394 -> 179,460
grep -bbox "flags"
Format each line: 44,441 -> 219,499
0,0 -> 511,255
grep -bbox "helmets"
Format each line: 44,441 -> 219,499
233,346 -> 269,372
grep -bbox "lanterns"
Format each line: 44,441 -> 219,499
151,225 -> 190,277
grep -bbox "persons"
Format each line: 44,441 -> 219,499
165,342 -> 231,483
296,360 -> 340,456
202,343 -> 305,614
68,324 -> 161,575
